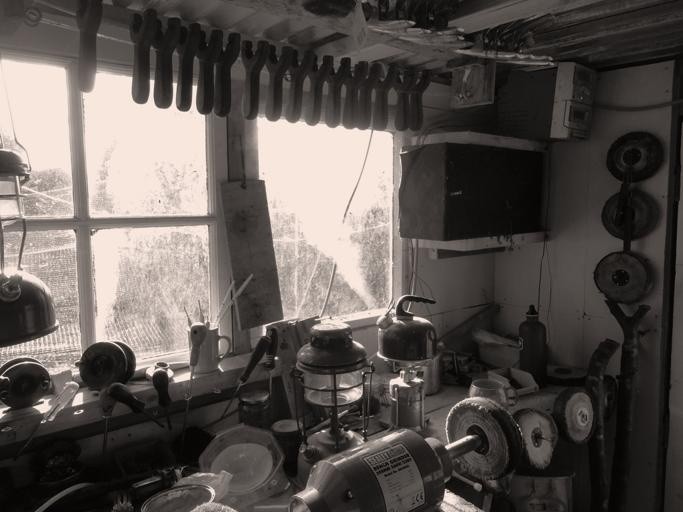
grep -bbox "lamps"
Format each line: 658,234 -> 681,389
0,59 -> 59,348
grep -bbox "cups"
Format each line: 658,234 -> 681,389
469,378 -> 519,412
187,326 -> 231,374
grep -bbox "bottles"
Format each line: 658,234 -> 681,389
270,419 -> 304,475
516,303 -> 551,387
236,388 -> 272,430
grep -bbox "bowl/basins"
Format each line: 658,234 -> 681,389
197,424 -> 283,499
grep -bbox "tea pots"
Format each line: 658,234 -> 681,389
376,293 -> 440,362
0,266 -> 59,349
